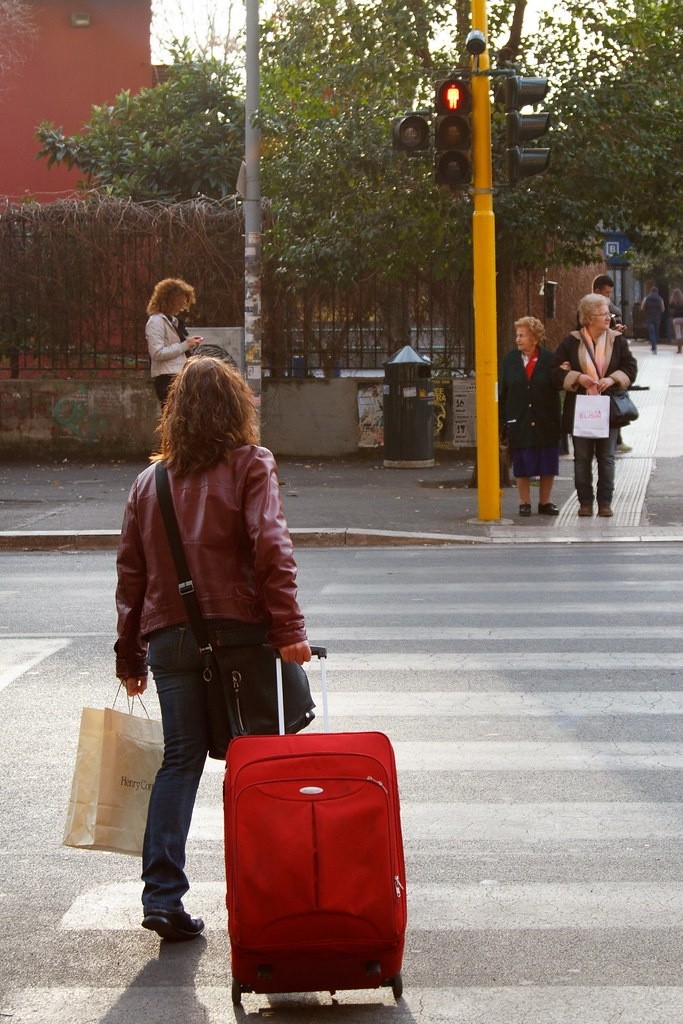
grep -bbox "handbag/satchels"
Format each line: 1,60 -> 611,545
62,676 -> 165,856
602,386 -> 638,423
204,644 -> 316,761
572,381 -> 610,439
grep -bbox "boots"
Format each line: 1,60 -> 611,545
677,339 -> 682,353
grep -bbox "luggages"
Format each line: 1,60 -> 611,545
223,646 -> 407,1005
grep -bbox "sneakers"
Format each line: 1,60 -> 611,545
614,442 -> 633,453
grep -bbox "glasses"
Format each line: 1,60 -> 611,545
590,313 -> 611,318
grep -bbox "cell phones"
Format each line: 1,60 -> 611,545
621,325 -> 626,329
197,337 -> 205,341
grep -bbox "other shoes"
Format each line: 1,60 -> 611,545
141,911 -> 204,939
598,503 -> 613,516
651,349 -> 657,355
578,504 -> 592,516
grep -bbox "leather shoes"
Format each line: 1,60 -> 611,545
538,503 -> 559,515
519,503 -> 531,516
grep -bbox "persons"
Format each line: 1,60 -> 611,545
553,274 -> 638,517
145,278 -> 202,411
114,355 -> 312,939
668,289 -> 683,354
498,316 -> 571,516
639,287 -> 665,355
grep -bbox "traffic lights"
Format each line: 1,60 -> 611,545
500,75 -> 552,186
394,79 -> 473,188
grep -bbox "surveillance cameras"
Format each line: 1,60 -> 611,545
465,31 -> 486,54
